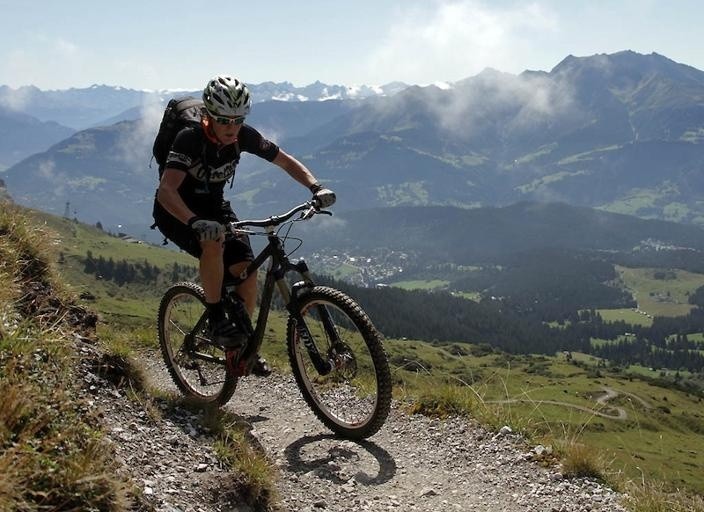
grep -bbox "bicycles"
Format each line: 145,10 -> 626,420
157,198 -> 393,440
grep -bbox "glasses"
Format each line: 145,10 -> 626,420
205,106 -> 246,125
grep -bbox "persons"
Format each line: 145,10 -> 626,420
153,74 -> 336,378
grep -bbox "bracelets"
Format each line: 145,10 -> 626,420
187,216 -> 202,229
310,182 -> 324,195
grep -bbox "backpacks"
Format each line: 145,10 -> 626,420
153,97 -> 214,166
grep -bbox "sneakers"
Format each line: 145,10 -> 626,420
249,355 -> 271,376
205,319 -> 246,347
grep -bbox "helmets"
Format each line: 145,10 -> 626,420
203,77 -> 251,116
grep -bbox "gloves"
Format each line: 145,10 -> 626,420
188,217 -> 225,243
310,181 -> 335,212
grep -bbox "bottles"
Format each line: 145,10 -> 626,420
227,287 -> 255,337
